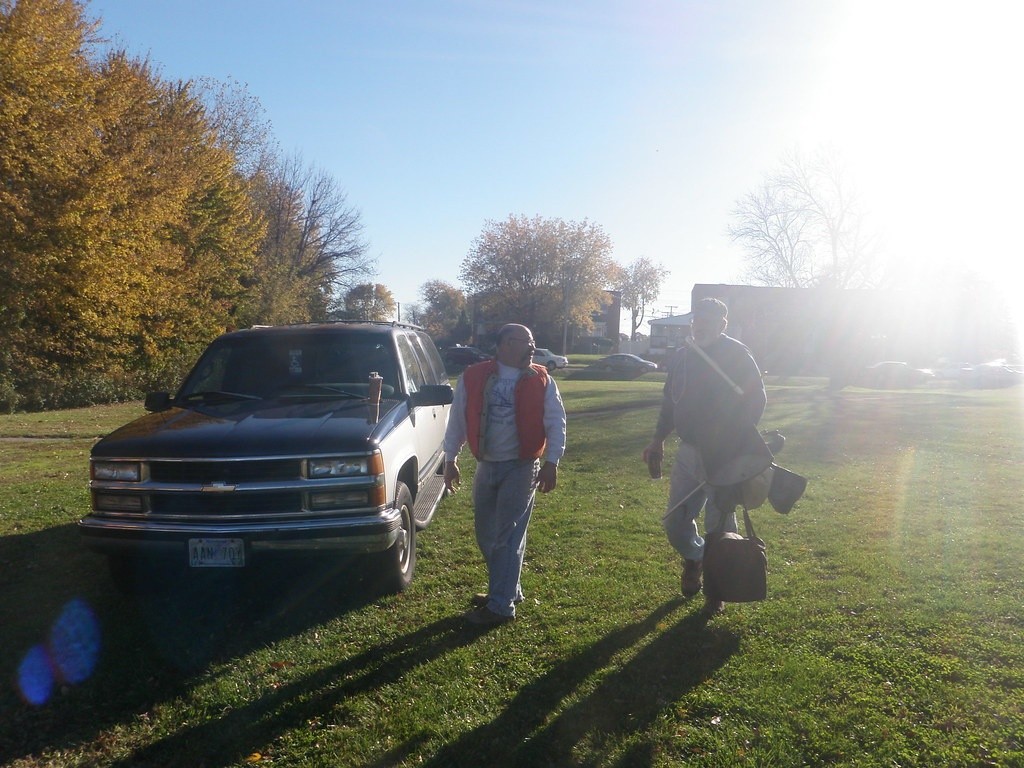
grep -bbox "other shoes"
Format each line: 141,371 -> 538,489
470,591 -> 525,608
702,600 -> 725,616
465,606 -> 516,624
681,546 -> 703,598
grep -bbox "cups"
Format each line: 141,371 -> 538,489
647,452 -> 662,480
369,376 -> 383,404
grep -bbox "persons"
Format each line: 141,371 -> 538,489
642,299 -> 768,611
443,324 -> 566,619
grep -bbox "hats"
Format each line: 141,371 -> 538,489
687,298 -> 728,317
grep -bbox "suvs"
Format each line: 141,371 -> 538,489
75,321 -> 454,592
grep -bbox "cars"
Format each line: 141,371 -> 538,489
441,347 -> 491,369
933,355 -> 1023,386
863,361 -> 935,386
532,349 -> 568,370
592,353 -> 658,372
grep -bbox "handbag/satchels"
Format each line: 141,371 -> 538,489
704,506 -> 766,603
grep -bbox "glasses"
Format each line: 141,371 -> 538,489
514,339 -> 536,347
